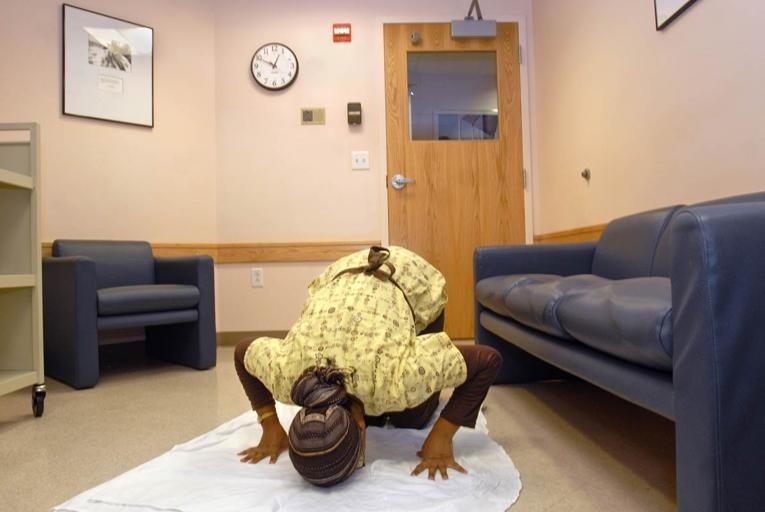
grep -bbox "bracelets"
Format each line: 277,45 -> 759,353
256,411 -> 277,424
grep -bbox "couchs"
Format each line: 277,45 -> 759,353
471,189 -> 764,512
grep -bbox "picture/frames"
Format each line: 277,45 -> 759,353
61,2 -> 154,128
653,0 -> 697,31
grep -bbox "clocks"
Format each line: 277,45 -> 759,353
250,41 -> 299,91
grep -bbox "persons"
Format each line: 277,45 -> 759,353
231,244 -> 504,490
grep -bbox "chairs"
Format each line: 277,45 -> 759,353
43,238 -> 216,389
